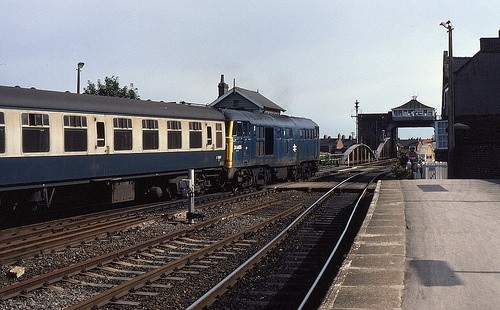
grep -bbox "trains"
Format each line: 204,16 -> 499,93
0,88 -> 320,228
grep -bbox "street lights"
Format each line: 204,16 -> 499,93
77,63 -> 84,94
